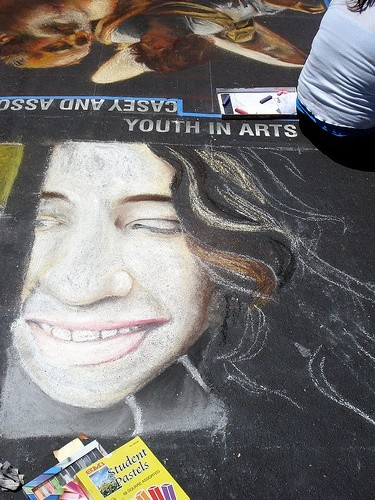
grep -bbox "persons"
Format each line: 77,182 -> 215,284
294,0 -> 375,167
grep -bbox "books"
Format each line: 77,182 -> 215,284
75,435 -> 192,500
